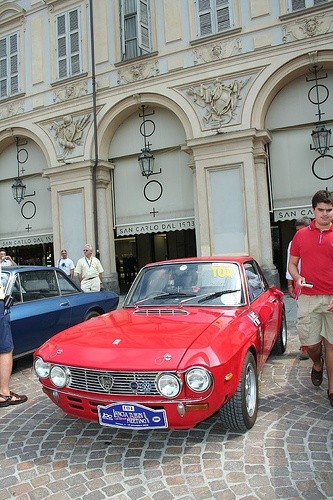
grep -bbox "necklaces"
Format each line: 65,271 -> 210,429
85,256 -> 92,268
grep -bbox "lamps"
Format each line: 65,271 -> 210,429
11,136 -> 27,204
137,104 -> 156,180
310,65 -> 332,158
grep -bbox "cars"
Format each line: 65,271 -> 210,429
32,255 -> 288,434
1,265 -> 120,358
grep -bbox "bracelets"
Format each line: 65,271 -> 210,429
288,285 -> 293,287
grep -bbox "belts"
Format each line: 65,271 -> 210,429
86,277 -> 96,280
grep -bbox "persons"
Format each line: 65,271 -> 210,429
0,280 -> 28,407
0,250 -> 18,292
74,244 -> 105,292
57,250 -> 75,290
286,190 -> 333,406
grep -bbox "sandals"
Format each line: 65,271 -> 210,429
0,391 -> 27,407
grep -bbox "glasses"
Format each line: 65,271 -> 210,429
61,249 -> 67,252
83,249 -> 88,252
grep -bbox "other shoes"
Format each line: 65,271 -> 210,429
300,350 -> 310,360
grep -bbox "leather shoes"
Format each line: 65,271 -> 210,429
327,389 -> 333,407
311,357 -> 324,386
